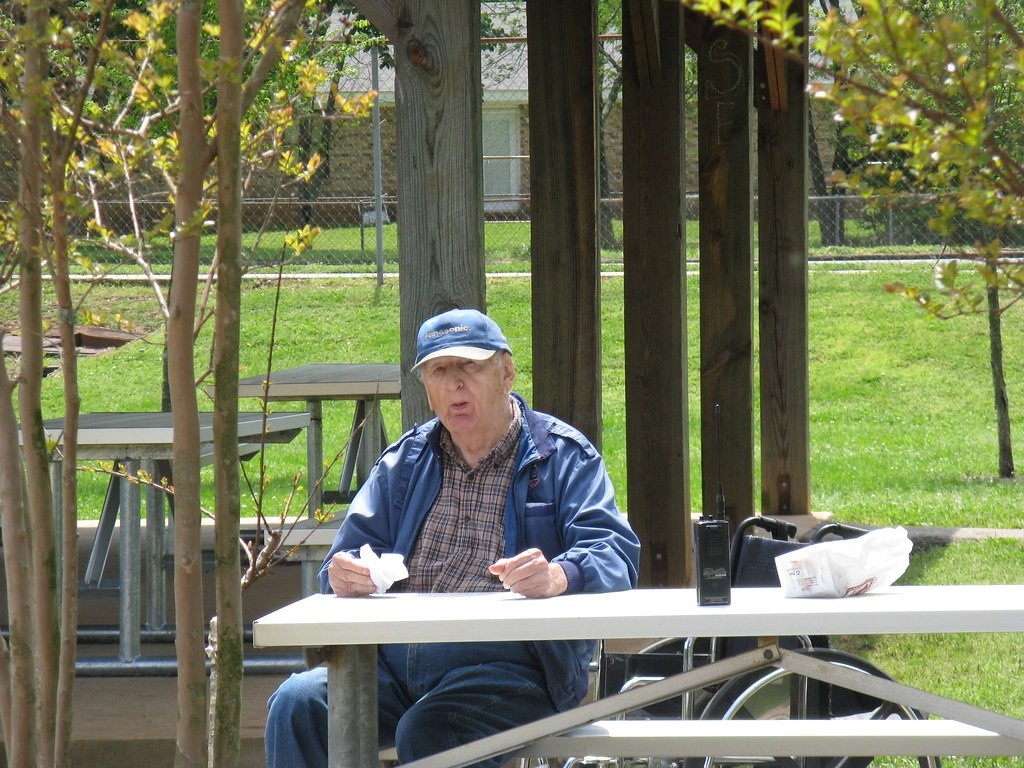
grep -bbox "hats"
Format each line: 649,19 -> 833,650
410,309 -> 513,370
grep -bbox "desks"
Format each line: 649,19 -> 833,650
252,583 -> 1024,768
207,363 -> 401,518
19,411 -> 310,677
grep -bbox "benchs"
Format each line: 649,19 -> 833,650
516,720 -> 1024,756
263,509 -> 351,598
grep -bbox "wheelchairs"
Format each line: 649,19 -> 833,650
518,516 -> 944,768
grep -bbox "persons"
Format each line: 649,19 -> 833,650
265,307 -> 641,768
